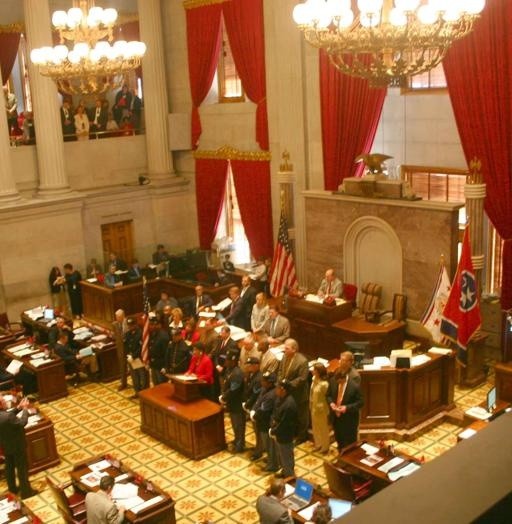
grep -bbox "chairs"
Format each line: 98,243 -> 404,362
354,280 -> 383,319
342,284 -> 358,312
45,475 -> 87,524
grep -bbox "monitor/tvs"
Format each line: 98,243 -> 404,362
344,341 -> 372,370
389,349 -> 412,369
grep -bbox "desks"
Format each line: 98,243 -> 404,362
0,308 -> 122,405
457,361 -> 512,442
262,438 -> 426,524
68,453 -> 176,524
0,490 -> 45,524
264,291 -> 406,362
0,391 -> 61,477
78,264 -> 260,330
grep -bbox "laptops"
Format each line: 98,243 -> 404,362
280,478 -> 314,512
328,497 -> 352,522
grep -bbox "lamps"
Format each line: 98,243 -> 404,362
292,0 -> 485,88
30,0 -> 147,98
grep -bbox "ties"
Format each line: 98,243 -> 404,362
335,383 -> 343,418
269,320 -> 275,337
199,296 -> 202,308
327,283 -> 332,295
221,342 -> 224,349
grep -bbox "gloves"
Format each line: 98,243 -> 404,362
126,355 -> 132,363
268,427 -> 278,441
250,410 -> 256,420
242,402 -> 249,410
219,395 -> 227,404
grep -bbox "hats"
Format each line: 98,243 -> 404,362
263,370 -> 277,383
225,348 -> 239,361
280,379 -> 292,391
244,358 -> 259,364
149,317 -> 160,324
126,319 -> 136,325
171,328 -> 182,336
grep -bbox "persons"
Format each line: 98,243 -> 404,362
307,350 -> 364,454
111,276 -> 309,481
246,255 -> 267,293
55,332 -> 86,374
105,251 -> 143,288
2,80 -> 144,146
317,269 -> 343,298
84,257 -> 102,281
63,262 -> 85,320
83,475 -> 126,524
222,255 -> 234,271
49,318 -> 69,349
303,505 -> 335,523
1,391 -> 43,498
152,245 -> 170,264
256,481 -> 292,524
48,266 -> 66,308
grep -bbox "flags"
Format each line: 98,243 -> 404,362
419,253 -> 454,350
267,189 -> 300,299
439,215 -> 485,349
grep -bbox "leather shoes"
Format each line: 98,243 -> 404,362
20,490 -> 38,499
295,439 -> 303,445
118,384 -> 126,391
231,440 -> 286,478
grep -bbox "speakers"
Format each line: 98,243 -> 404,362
167,113 -> 192,151
